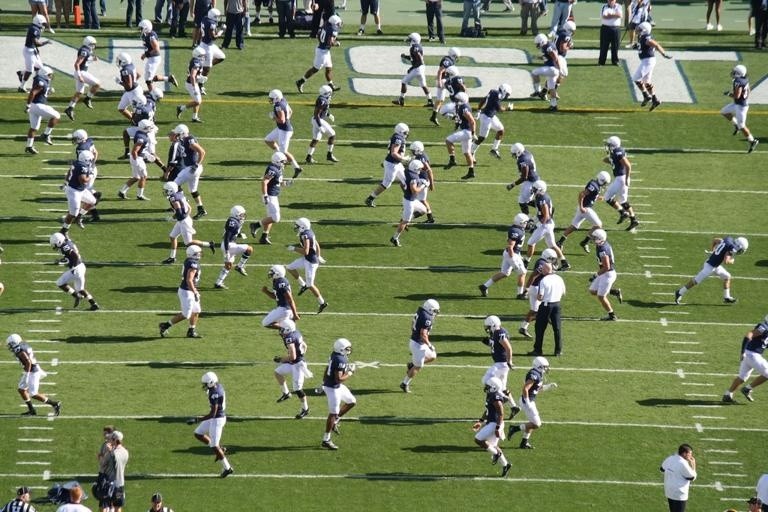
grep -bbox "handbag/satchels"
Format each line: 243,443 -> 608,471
92,483 -> 114,498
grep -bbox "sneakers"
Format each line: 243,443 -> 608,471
392,99 -> 403,105
517,294 -> 528,300
296,79 -> 305,93
479,283 -> 489,297
400,363 -> 413,393
531,88 -> 558,111
214,448 -> 233,478
430,115 -> 440,125
118,189 -> 149,201
117,151 -> 131,159
318,303 -> 327,313
579,241 -> 591,253
177,105 -> 203,123
556,241 -> 571,270
444,149 -> 501,178
21,410 -> 35,415
214,266 -> 246,289
674,289 -> 681,303
616,212 -> 638,231
74,294 -> 98,311
297,409 -> 340,450
365,197 -> 435,247
600,289 -> 622,320
331,84 -> 340,91
723,298 -> 737,303
722,387 -> 754,403
492,406 -> 532,477
60,214 -> 99,240
159,321 -> 201,339
642,95 -> 659,110
733,124 -> 758,154
168,74 -> 177,85
53,401 -> 61,416
298,285 -> 307,295
249,222 -> 269,245
277,393 -> 291,402
26,107 -> 75,154
17,70 -> 28,92
83,95 -> 93,108
292,152 -> 338,177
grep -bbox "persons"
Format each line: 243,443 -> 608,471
660,443 -> 697,512
674,236 -> 749,305
756,473 -> 768,512
748,0 -> 767,50
0,1 -> 672,512
719,65 -> 759,153
746,497 -> 762,512
723,314 -> 768,404
705,0 -> 723,31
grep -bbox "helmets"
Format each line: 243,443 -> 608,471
499,82 -> 513,100
637,21 -> 651,35
192,9 -> 221,62
269,264 -> 296,336
407,32 -> 468,105
328,15 -> 342,27
32,14 -> 97,80
164,181 -> 246,259
173,123 -> 188,139
333,338 -> 351,356
395,122 -> 425,174
534,22 -> 575,48
295,218 -> 312,233
7,334 -> 21,349
423,298 -> 440,316
510,143 -> 557,264
735,236 -> 749,252
73,130 -> 94,164
484,315 -> 549,395
271,152 -> 286,168
50,232 -> 64,248
592,229 -> 607,245
597,135 -> 620,185
320,85 -> 332,98
734,65 -> 747,78
115,19 -> 164,135
201,372 -> 218,391
268,89 -> 282,104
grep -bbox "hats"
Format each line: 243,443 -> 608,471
105,431 -> 124,441
